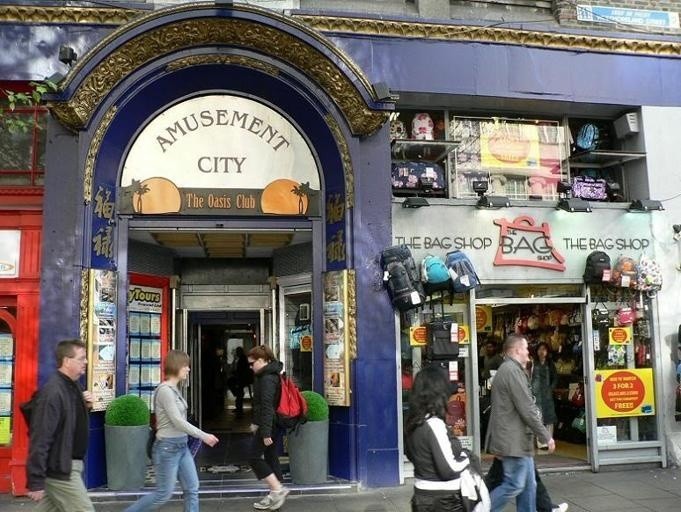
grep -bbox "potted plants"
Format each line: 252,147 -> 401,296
104,394 -> 150,490
286,391 -> 329,485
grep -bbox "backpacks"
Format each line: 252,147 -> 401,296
583,251 -> 662,292
380,244 -> 424,311
421,254 -> 452,293
447,251 -> 477,293
262,371 -> 308,429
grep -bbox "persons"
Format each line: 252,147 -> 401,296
405,366 -> 472,512
244,345 -> 289,510
125,350 -> 221,512
481,332 -> 568,512
227,346 -> 251,412
25,345 -> 96,511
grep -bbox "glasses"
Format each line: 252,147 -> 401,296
249,359 -> 258,366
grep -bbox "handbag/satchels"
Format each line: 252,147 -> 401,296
390,120 -> 406,151
577,122 -> 600,149
555,358 -> 576,375
572,175 -> 608,201
591,308 -> 652,368
391,161 -> 447,191
148,413 -> 196,461
444,400 -> 465,426
571,383 -> 586,434
413,113 -> 435,141
518,309 -> 582,352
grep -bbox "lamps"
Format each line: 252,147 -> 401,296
372,81 -> 391,102
556,198 -> 592,213
402,197 -> 430,208
629,199 -> 665,211
59,46 -> 77,67
477,195 -> 512,208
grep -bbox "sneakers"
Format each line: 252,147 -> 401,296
253,483 -> 290,511
551,503 -> 568,512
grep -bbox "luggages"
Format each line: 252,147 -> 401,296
425,298 -> 459,364
